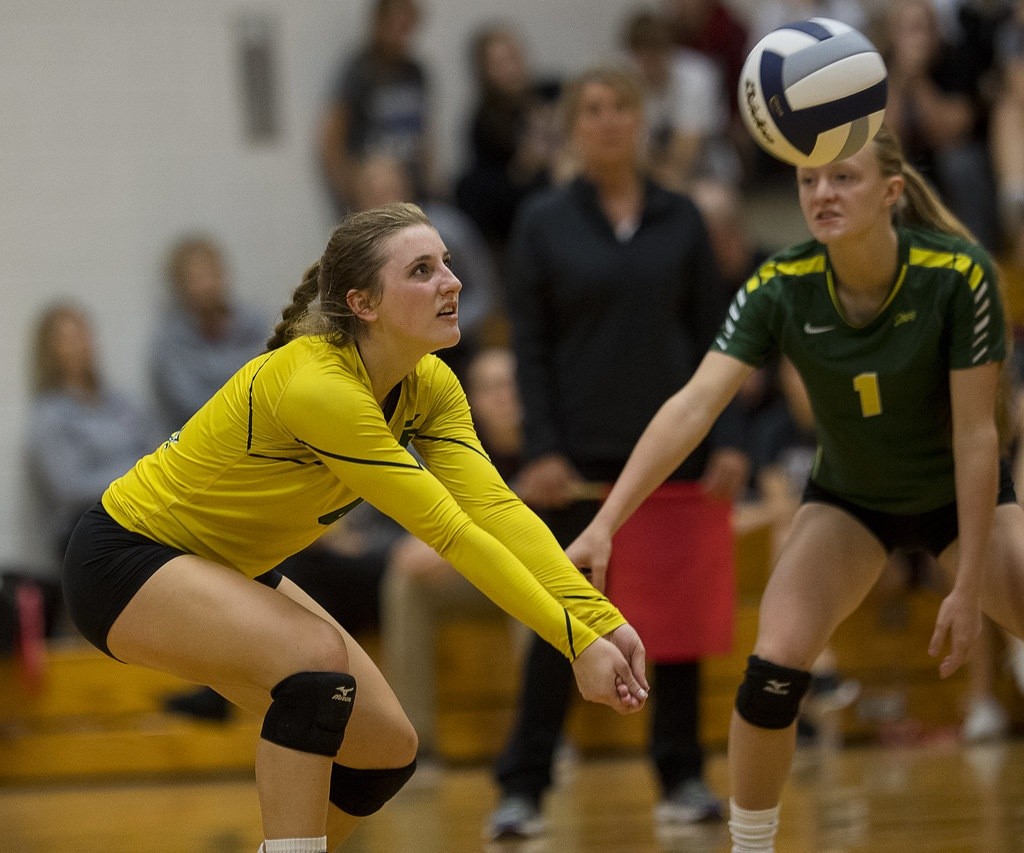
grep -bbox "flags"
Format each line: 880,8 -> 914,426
606,483 -> 732,661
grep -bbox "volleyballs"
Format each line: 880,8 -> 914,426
734,16 -> 888,170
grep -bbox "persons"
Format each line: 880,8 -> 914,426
147,0 -> 1024,853
61,203 -> 649,853
18,299 -> 160,548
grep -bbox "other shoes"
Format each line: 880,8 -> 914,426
652,778 -> 722,825
483,795 -> 544,842
789,711 -> 822,749
961,704 -> 1004,740
814,670 -> 859,714
